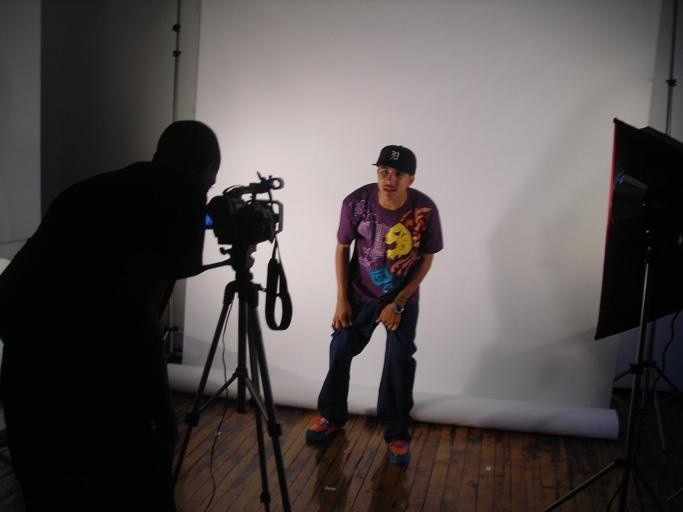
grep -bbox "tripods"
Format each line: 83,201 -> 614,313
173,245 -> 297,512
541,265 -> 671,512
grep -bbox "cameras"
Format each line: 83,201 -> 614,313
207,174 -> 284,241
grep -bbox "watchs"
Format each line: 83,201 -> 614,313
391,300 -> 407,315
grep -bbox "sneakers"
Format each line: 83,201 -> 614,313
387,440 -> 410,463
305,418 -> 346,437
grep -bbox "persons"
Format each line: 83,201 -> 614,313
0,116 -> 223,511
304,143 -> 445,465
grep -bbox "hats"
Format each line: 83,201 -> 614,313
372,145 -> 416,175
